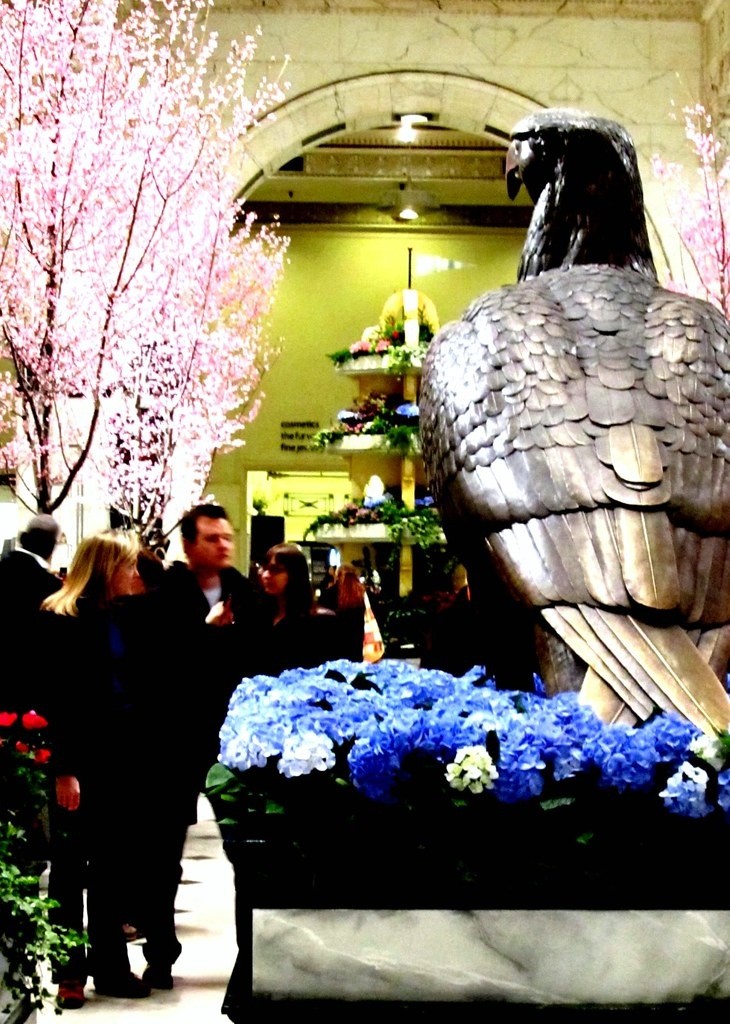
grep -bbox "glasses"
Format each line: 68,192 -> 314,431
262,565 -> 285,575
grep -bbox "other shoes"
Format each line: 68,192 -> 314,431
122,923 -> 138,941
142,961 -> 173,989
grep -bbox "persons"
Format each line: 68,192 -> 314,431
0,502 -> 369,1009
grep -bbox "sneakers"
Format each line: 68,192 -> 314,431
57,977 -> 84,1009
94,973 -> 151,997
